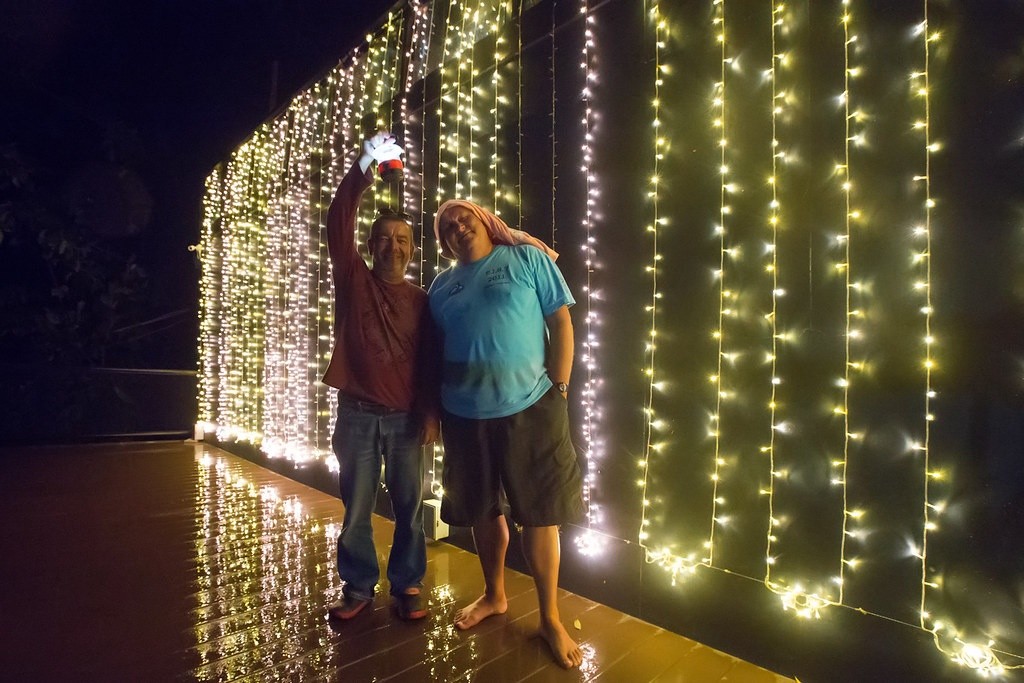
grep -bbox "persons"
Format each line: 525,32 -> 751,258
322,127 -> 442,629
423,196 -> 588,670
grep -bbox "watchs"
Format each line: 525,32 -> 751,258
555,381 -> 570,393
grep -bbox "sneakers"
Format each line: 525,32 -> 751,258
391,585 -> 427,619
328,593 -> 368,620
